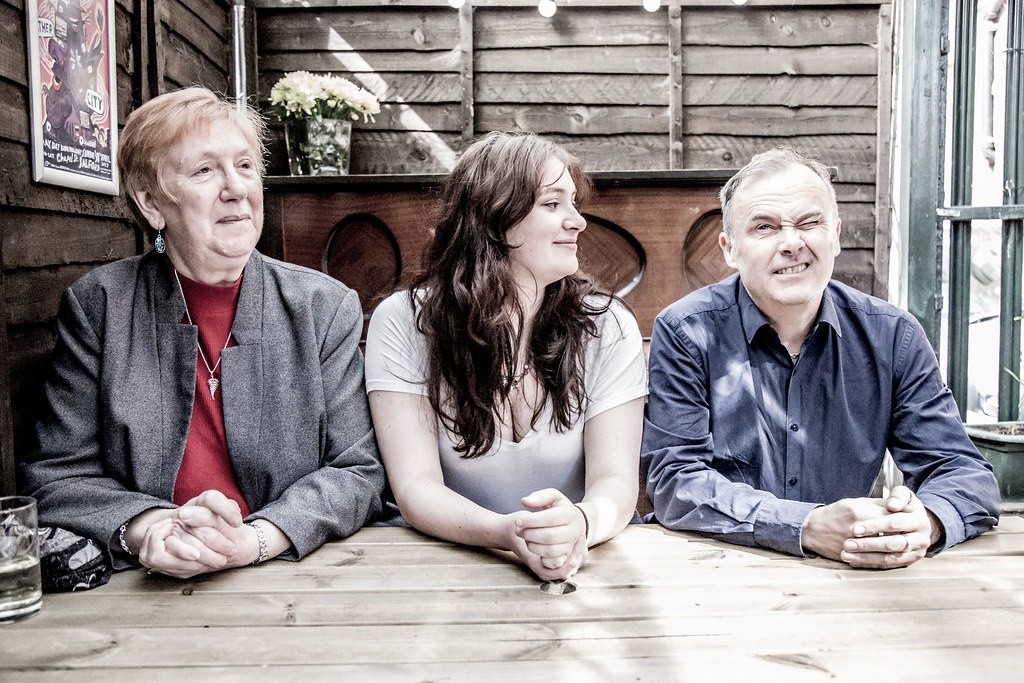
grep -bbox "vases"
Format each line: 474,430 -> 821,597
284,117 -> 353,176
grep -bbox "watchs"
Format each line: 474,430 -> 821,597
243,522 -> 269,566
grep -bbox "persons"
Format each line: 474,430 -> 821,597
365,131 -> 651,585
640,144 -> 1000,569
20,90 -> 386,579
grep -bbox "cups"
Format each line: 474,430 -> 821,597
0,496 -> 43,623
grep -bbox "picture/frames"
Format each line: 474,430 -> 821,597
24,0 -> 121,196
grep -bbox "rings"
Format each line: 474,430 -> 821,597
145,566 -> 161,577
899,533 -> 913,554
878,532 -> 885,536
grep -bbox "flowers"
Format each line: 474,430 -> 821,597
267,70 -> 381,125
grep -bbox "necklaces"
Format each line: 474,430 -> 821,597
499,351 -> 532,390
791,352 -> 799,359
174,269 -> 232,401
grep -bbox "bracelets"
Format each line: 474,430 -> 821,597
115,523 -> 138,556
573,504 -> 589,540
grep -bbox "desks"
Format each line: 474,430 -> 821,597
0,510 -> 1024,683
